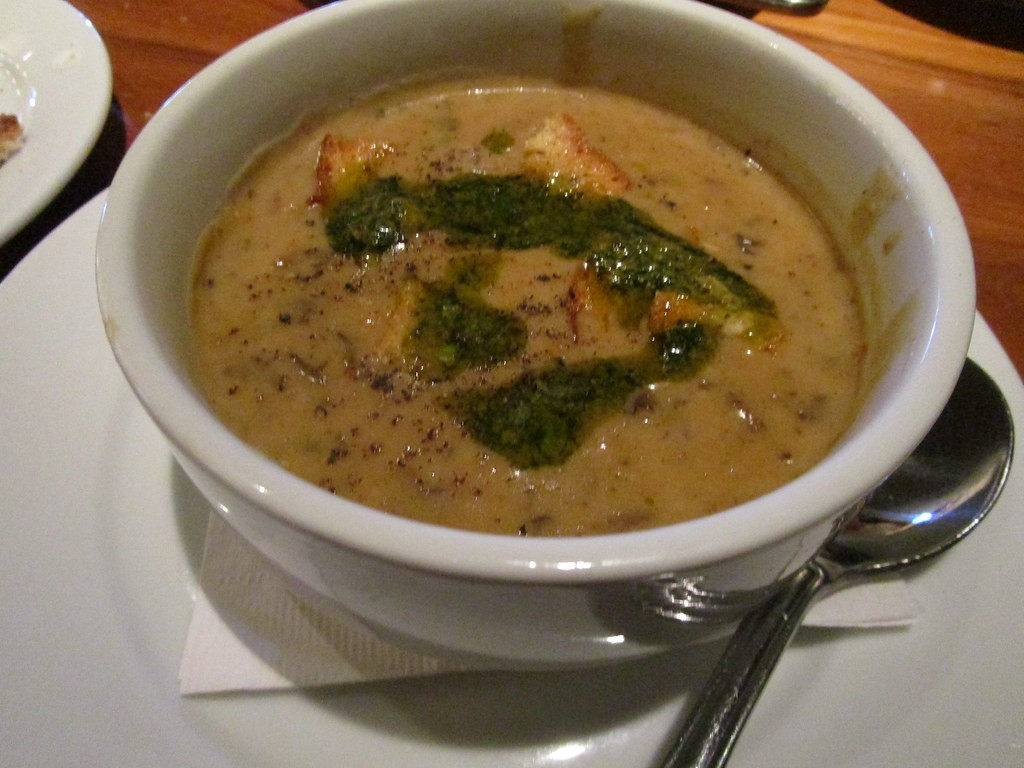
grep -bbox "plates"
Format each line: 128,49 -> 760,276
0,2 -> 111,245
0,191 -> 1023,768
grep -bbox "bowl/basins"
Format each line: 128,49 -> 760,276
92,2 -> 974,666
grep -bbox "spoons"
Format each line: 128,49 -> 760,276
652,357 -> 1015,768
